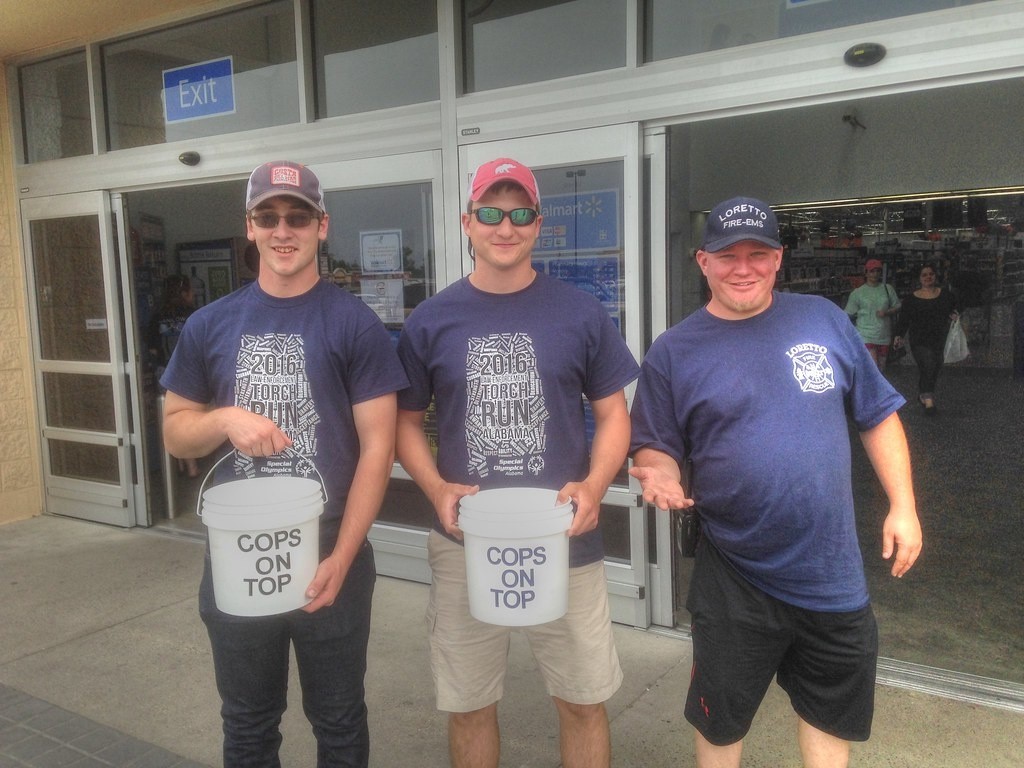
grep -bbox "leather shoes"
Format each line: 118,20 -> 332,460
918,396 -> 937,414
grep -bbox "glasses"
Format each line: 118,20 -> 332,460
247,212 -> 319,228
469,206 -> 539,226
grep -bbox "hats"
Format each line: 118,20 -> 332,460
704,196 -> 780,253
469,158 -> 540,212
245,161 -> 325,216
865,260 -> 883,270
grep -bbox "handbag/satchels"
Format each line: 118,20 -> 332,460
942,315 -> 970,363
886,327 -> 906,364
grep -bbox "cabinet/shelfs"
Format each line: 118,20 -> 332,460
784,257 -> 934,310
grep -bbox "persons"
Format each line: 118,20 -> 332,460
626,198 -> 923,768
391,158 -> 643,768
158,160 -> 409,767
843,261 -> 901,377
893,266 -> 961,415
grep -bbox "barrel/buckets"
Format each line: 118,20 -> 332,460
459,487 -> 574,626
197,445 -> 328,617
